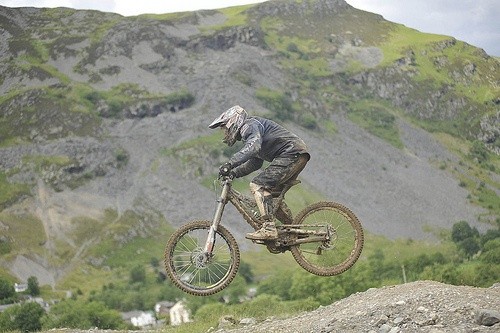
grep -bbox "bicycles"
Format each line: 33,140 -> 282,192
164,167 -> 365,296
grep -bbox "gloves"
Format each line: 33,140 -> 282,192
219,162 -> 231,177
227,172 -> 236,180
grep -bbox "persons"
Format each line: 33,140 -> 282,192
208,105 -> 312,243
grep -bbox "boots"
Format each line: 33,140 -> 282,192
245,182 -> 278,240
272,196 -> 309,239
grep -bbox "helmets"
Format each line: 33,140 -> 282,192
207,105 -> 248,146
